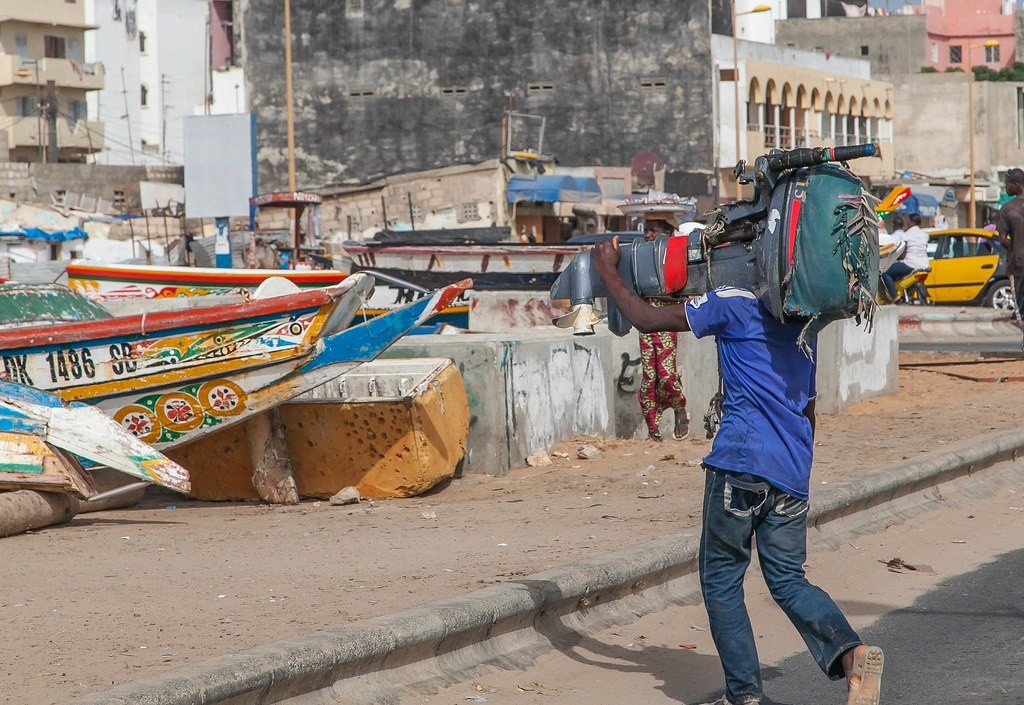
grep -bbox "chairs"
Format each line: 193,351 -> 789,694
953,241 -> 963,257
975,242 -> 991,256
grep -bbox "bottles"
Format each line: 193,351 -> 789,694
530,225 -> 536,243
520,225 -> 528,243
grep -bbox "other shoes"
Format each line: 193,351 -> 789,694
894,297 -> 903,304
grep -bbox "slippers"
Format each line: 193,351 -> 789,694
673,410 -> 690,440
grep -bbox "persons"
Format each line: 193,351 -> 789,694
592,236 -> 885,704
995,168 -> 1024,353
637,212 -> 691,443
878,214 -> 933,306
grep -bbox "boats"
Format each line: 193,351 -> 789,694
0,226 -> 640,502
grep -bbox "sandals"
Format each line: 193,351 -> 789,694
846,645 -> 884,705
701,694 -> 758,705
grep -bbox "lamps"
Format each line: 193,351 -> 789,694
860,83 -> 870,89
839,80 -> 846,83
826,78 -> 837,83
885,87 -> 892,92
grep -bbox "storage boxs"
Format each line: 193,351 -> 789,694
160,356 -> 471,502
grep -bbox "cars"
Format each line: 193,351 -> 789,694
909,228 -> 1015,311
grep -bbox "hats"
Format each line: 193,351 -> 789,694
643,209 -> 680,232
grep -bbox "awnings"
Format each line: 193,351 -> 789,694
508,174 -> 602,219
895,194 -> 938,217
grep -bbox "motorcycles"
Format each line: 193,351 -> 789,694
874,267 -> 935,305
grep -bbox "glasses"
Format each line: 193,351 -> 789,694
1005,168 -> 1013,178
